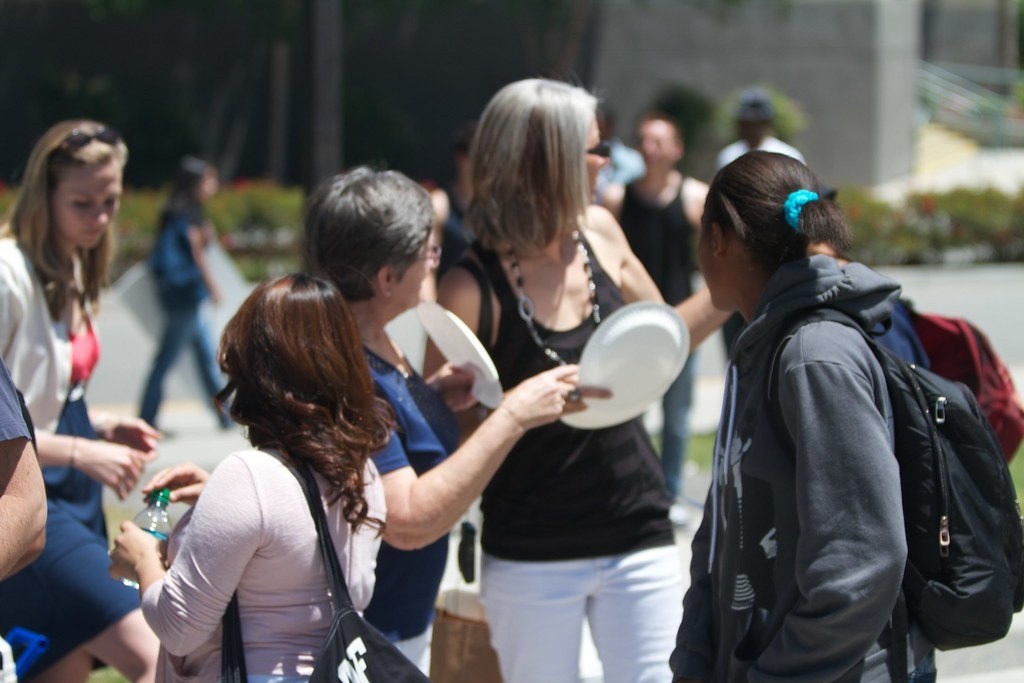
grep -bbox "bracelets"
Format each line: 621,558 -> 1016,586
69,436 -> 77,465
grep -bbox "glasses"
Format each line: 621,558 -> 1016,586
579,138 -> 611,158
414,243 -> 442,268
51,124 -> 120,158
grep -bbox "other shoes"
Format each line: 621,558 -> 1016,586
153,428 -> 175,440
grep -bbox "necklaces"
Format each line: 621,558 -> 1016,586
504,225 -> 601,365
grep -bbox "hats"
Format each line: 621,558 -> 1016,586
738,90 -> 774,126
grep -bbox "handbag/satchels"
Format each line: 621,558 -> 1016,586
308,602 -> 429,683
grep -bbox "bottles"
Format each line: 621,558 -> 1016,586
121,488 -> 172,590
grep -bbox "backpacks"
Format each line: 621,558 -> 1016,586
762,307 -> 1023,648
895,290 -> 1023,461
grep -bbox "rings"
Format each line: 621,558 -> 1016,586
568,387 -> 581,403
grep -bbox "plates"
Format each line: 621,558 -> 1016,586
415,298 -> 502,409
559,302 -> 692,430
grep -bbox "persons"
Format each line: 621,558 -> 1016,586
668,151 -> 937,683
0,357 -> 47,683
139,157 -> 235,439
422,77 -> 847,683
600,112 -> 711,526
592,103 -> 646,205
713,94 -> 807,177
300,166 -> 614,683
0,119 -> 164,683
107,273 -> 388,683
